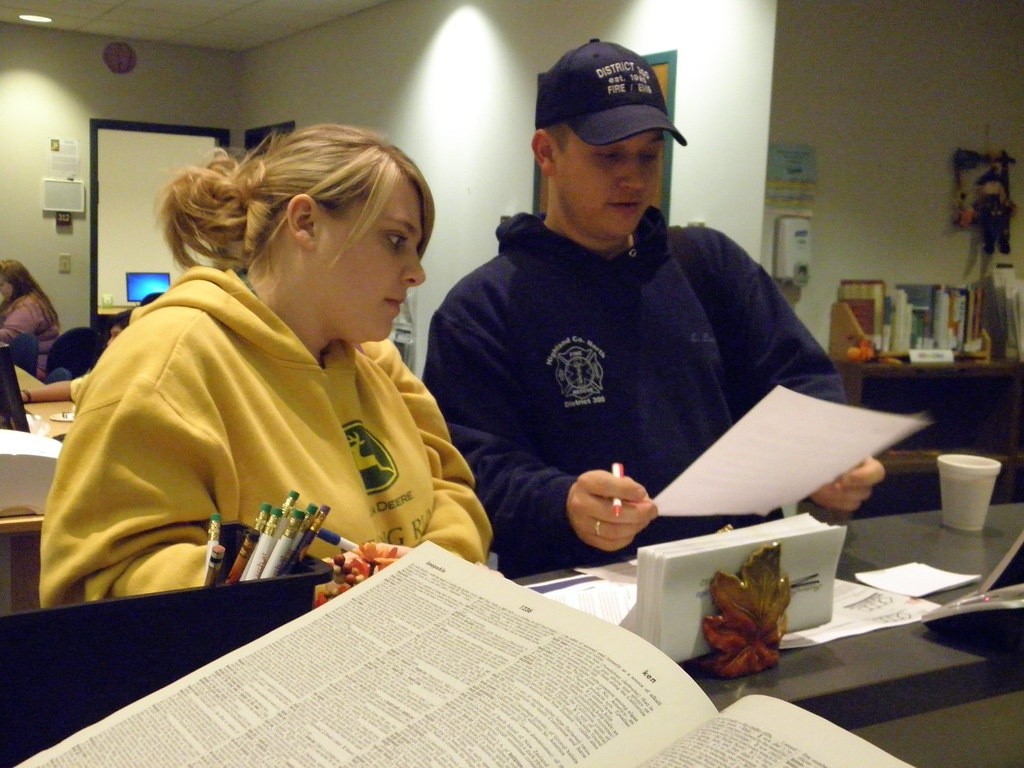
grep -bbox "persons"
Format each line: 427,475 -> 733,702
39,125 -> 493,595
976,170 -> 1016,254
0,260 -> 162,404
423,38 -> 887,583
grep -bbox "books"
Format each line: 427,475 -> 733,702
836,280 -> 1024,363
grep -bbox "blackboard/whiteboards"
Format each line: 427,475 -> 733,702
43,178 -> 84,213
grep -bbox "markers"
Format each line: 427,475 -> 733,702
54,178 -> 74,182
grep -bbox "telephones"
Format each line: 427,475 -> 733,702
921,528 -> 1024,640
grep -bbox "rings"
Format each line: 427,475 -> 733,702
596,520 -> 601,537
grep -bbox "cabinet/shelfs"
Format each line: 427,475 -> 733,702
833,360 -> 1024,503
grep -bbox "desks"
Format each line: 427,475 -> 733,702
0,364 -> 74,437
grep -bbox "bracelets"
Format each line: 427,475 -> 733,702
20,388 -> 33,404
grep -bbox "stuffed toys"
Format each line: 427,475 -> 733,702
959,194 -> 975,228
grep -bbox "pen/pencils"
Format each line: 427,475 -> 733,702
205,490 -> 333,587
611,462 -> 624,521
314,528 -> 362,551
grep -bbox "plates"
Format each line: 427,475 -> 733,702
49,414 -> 73,422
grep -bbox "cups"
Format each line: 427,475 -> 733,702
938,455 -> 1001,531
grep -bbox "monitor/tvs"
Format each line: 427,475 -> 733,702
126,272 -> 170,302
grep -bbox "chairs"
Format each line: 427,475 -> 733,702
8,332 -> 40,377
45,327 -> 106,380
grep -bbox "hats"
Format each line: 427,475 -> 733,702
536,38 -> 688,146
